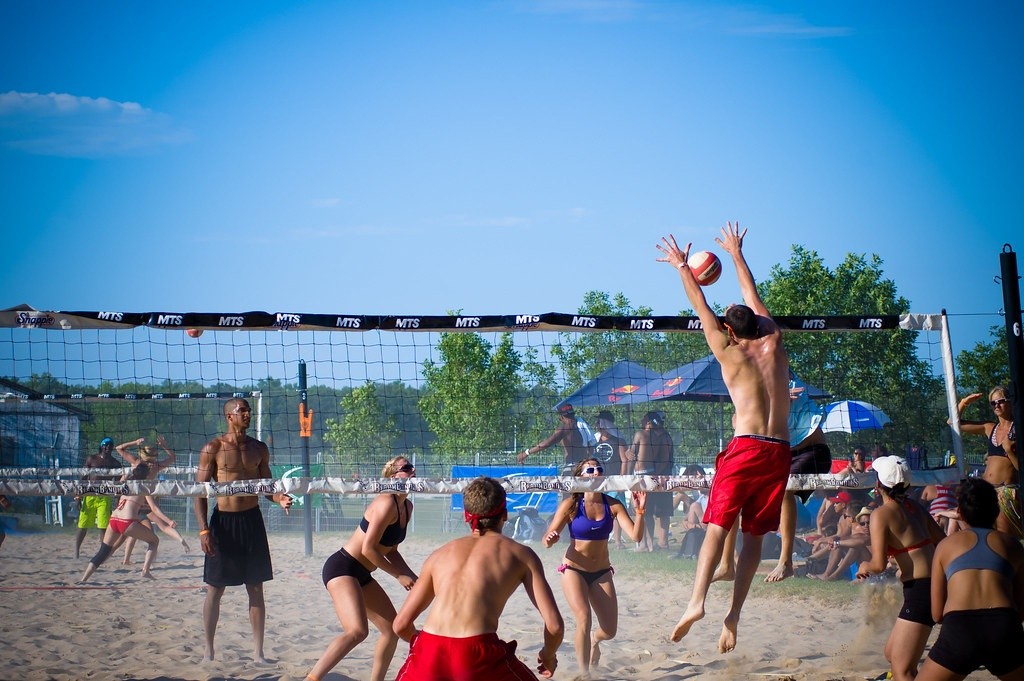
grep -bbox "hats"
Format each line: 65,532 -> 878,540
594,410 -> 615,423
101,438 -> 113,446
559,405 -> 574,415
872,455 -> 911,488
648,410 -> 665,426
829,492 -> 852,503
856,507 -> 873,523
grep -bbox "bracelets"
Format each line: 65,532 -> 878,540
200,530 -> 210,536
677,262 -> 688,270
636,508 -> 645,515
836,540 -> 840,548
962,399 -> 970,407
525,449 -> 530,456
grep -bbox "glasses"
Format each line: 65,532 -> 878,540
395,464 -> 413,474
854,453 -> 862,455
102,443 -> 113,446
835,502 -> 839,504
581,466 -> 603,476
991,398 -> 1011,406
859,521 -> 869,526
844,514 -> 852,519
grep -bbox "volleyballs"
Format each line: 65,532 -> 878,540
186,329 -> 203,338
688,250 -> 722,286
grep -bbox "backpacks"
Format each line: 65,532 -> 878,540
503,509 -> 570,542
678,528 -> 706,559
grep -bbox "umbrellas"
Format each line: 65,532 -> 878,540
818,399 -> 893,433
618,354 -> 833,454
552,360 -> 660,449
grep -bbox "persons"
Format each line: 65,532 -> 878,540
304,456 -> 420,681
518,364 -> 1024,586
116,437 -> 175,565
73,437 -> 124,560
192,398 -> 292,663
915,477 -> 1024,681
655,221 -> 791,654
82,463 -> 177,585
109,500 -> 191,556
543,457 -> 647,681
393,477 -> 564,681
855,455 -> 948,681
